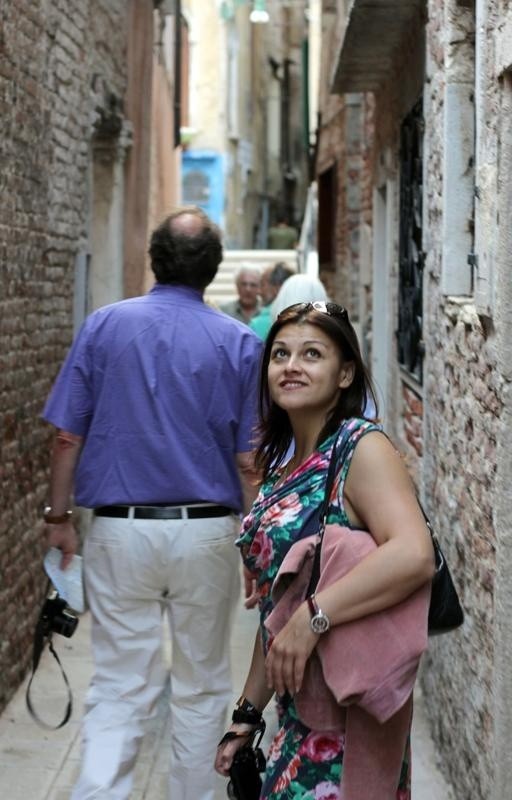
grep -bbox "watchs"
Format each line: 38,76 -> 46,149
308,594 -> 333,637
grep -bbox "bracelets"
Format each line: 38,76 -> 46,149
43,506 -> 72,524
232,698 -> 262,726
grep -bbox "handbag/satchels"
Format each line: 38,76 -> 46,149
304,422 -> 465,638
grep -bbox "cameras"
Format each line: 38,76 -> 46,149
229,748 -> 261,800
39,590 -> 78,638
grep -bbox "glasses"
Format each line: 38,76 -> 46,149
279,302 -> 347,316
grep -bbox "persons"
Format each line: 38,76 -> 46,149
218,260 -> 329,339
43,206 -> 264,800
214,302 -> 433,798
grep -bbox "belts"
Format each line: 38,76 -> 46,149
94,506 -> 232,520
